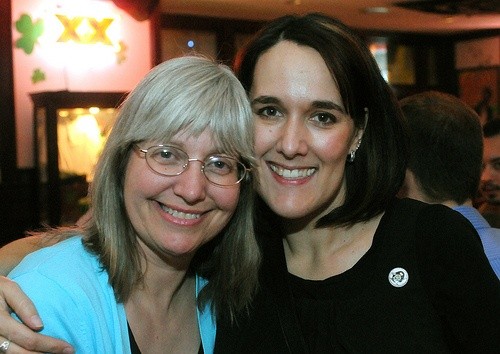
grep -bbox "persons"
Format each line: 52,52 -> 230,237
0,12 -> 500,354
0,55 -> 262,354
394,91 -> 500,283
475,118 -> 500,229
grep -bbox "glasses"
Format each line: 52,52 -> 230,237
132,142 -> 253,187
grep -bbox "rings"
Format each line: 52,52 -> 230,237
0,341 -> 10,354
